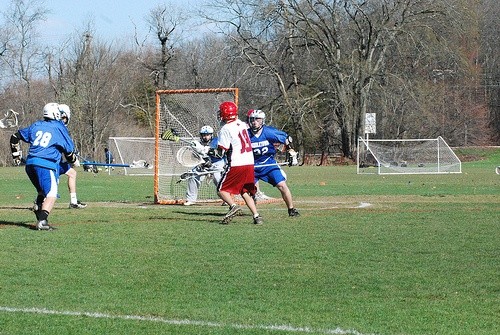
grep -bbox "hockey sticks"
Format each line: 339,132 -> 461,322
161,128 -> 196,146
177,147 -> 224,172
0,109 -> 20,129
80,159 -> 153,169
180,161 -> 289,182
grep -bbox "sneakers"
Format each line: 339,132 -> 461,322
67,202 -> 87,210
253,214 -> 265,225
287,208 -> 300,217
222,205 -> 240,223
37,220 -> 52,231
184,199 -> 195,206
220,200 -> 229,207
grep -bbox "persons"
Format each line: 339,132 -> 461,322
214,108 -> 300,217
184,125 -> 228,206
104,148 -> 115,171
286,142 -> 294,167
31,104 -> 87,212
192,100 -> 263,224
10,103 -> 81,230
83,153 -> 97,172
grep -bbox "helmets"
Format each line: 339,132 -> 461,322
199,126 -> 214,136
42,103 -> 61,120
219,101 -> 238,121
58,104 -> 71,125
247,109 -> 266,130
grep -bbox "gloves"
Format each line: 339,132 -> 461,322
289,152 -> 296,165
192,141 -> 209,155
74,160 -> 82,166
11,157 -> 20,165
